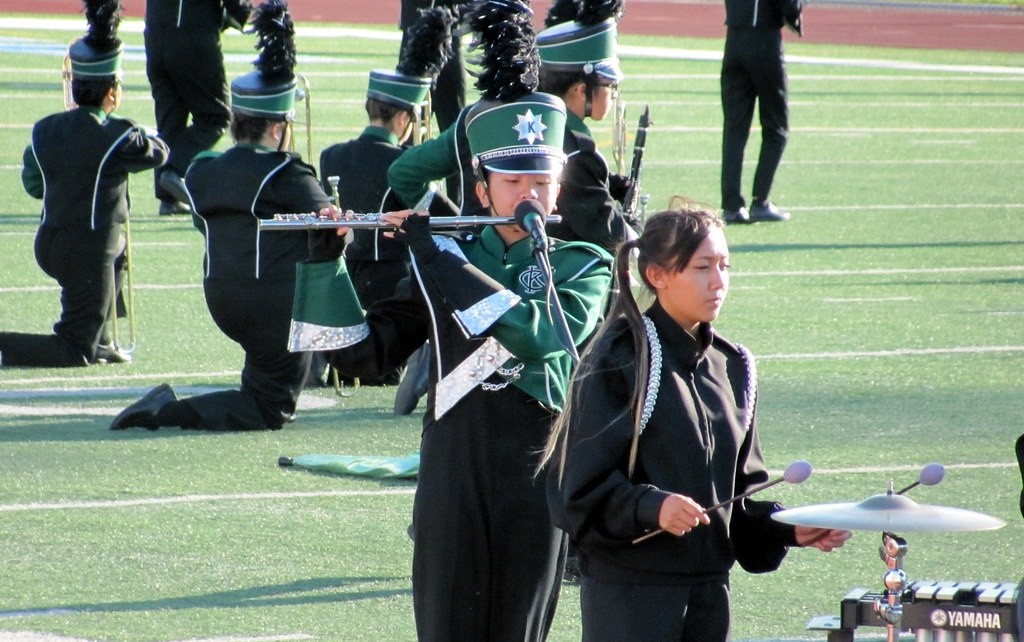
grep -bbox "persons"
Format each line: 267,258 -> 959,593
388,128 -> 457,415
290,92 -> 616,642
0,38 -> 171,369
109,71 -> 329,432
309,69 -> 432,387
537,194 -> 853,642
534,19 -> 641,584
145,0 -> 254,214
399,2 -> 471,205
721,0 -> 804,222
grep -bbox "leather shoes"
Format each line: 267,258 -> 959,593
750,203 -> 790,221
722,205 -> 757,224
109,382 -> 179,431
310,347 -> 331,389
159,169 -> 191,206
94,339 -> 132,365
394,342 -> 431,415
160,199 -> 191,215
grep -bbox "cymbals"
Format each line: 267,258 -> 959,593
768,489 -> 1006,533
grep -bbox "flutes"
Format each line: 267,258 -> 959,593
255,210 -> 563,229
620,104 -> 652,227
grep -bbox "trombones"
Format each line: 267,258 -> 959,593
288,71 -> 359,396
58,37 -> 136,356
411,83 -> 432,150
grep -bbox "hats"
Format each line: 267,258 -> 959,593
232,0 -> 297,123
367,0 -> 454,123
536,0 -> 627,81
69,0 -> 124,82
455,0 -> 567,174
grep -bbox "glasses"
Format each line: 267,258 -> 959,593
574,81 -> 621,92
